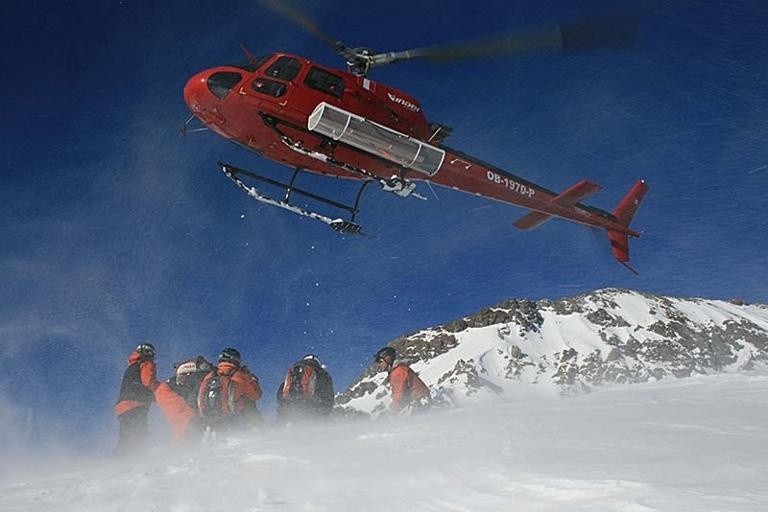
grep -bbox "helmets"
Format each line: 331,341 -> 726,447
135,343 -> 155,355
375,346 -> 397,363
303,353 -> 321,366
218,347 -> 241,367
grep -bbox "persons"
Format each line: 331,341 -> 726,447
276,355 -> 336,427
371,346 -> 432,419
112,341 -> 163,452
196,346 -> 265,441
154,356 -> 220,449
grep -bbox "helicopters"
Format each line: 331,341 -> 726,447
181,2 -> 650,276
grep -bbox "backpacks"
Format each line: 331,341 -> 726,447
199,366 -> 246,429
172,354 -> 216,386
288,362 -> 332,416
